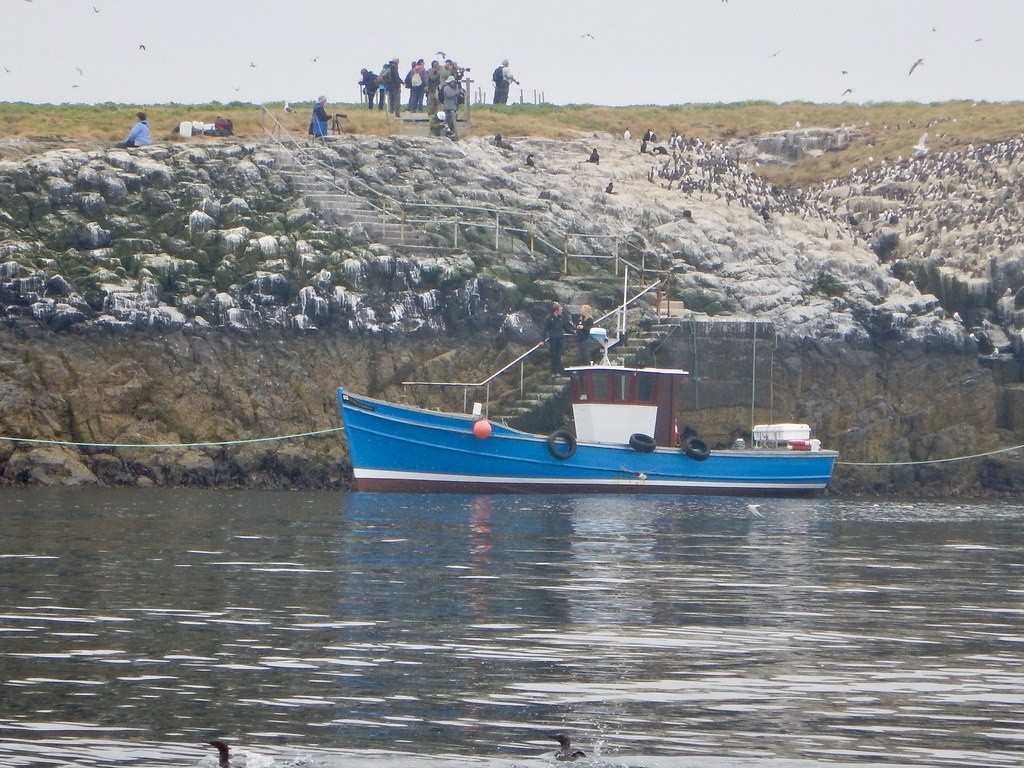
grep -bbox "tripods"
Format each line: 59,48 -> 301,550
332,116 -> 346,135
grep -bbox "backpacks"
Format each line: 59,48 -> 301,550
492,67 -> 504,83
382,66 -> 393,83
412,68 -> 423,87
438,85 -> 448,104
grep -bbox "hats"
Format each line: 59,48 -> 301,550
502,58 -> 509,65
392,56 -> 399,61
445,76 -> 455,83
437,112 -> 445,120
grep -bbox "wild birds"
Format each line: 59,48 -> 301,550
436,52 -> 446,59
526,154 -> 534,166
722,0 -> 983,96
548,733 -> 587,761
495,133 -> 501,146
606,182 -> 614,194
208,740 -> 231,768
4,0 -> 320,88
581,34 -> 594,40
623,104 -> 1023,253
283,101 -> 297,113
589,148 -> 599,163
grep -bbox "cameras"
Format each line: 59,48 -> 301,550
511,77 -> 519,85
334,113 -> 347,118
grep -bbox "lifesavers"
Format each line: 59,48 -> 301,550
683,437 -> 710,461
630,433 -> 655,452
548,430 -> 577,459
786,438 -> 822,452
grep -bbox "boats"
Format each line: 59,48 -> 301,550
337,264 -> 839,499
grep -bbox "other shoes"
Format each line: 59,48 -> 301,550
551,373 -> 560,379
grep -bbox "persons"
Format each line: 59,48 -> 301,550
573,304 -> 594,366
308,96 -> 337,138
357,57 -> 465,141
538,304 -> 576,378
492,59 -> 513,105
107,112 -> 150,149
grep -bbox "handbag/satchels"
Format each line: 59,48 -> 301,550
215,115 -> 233,131
